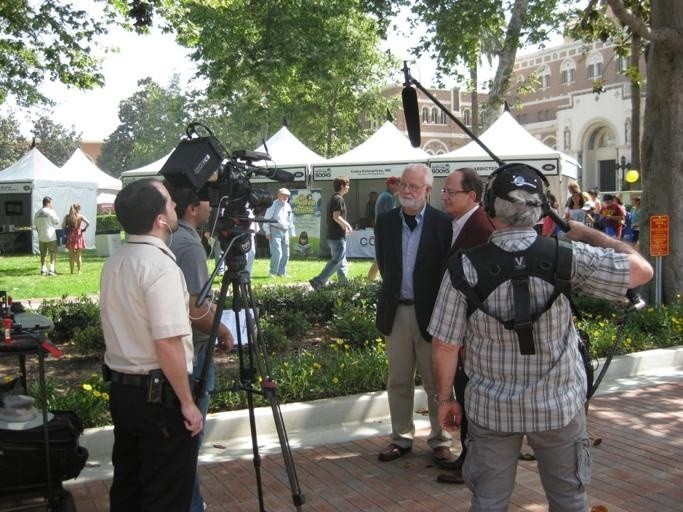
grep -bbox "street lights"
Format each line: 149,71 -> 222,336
624,169 -> 638,190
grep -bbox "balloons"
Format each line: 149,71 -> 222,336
626,169 -> 640,184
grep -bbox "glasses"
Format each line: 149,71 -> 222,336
398,181 -> 424,191
441,189 -> 471,196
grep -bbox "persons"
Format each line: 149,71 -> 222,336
34,196 -> 59,276
427,163 -> 654,512
295,232 -> 313,258
544,181 -> 643,246
161,177 -> 233,511
63,204 -> 90,274
309,173 -> 400,293
433,167 -> 495,485
100,178 -> 203,511
204,196 -> 260,285
374,164 -> 453,470
262,187 -> 296,279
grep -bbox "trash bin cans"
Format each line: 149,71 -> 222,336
95,229 -> 121,258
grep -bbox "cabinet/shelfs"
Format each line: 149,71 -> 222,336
0,308 -> 61,512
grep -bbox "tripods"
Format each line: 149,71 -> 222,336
194,232 -> 306,512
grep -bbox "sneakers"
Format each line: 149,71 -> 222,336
48,272 -> 56,276
309,280 -> 318,289
439,457 -> 463,471
432,446 -> 449,463
379,442 -> 411,461
437,473 -> 464,483
41,271 -> 47,275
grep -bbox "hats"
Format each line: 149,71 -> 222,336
387,177 -> 398,184
279,188 -> 291,196
0,395 -> 55,431
492,166 -> 543,206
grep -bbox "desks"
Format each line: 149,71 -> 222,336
0,231 -> 23,251
345,230 -> 376,259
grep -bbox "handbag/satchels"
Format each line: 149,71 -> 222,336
62,229 -> 67,245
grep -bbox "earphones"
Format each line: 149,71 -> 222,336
160,219 -> 166,224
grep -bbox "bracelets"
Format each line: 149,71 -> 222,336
433,393 -> 454,406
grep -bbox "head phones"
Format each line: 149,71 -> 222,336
481,163 -> 550,218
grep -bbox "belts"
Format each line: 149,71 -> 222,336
109,372 -> 151,388
398,300 -> 414,305
